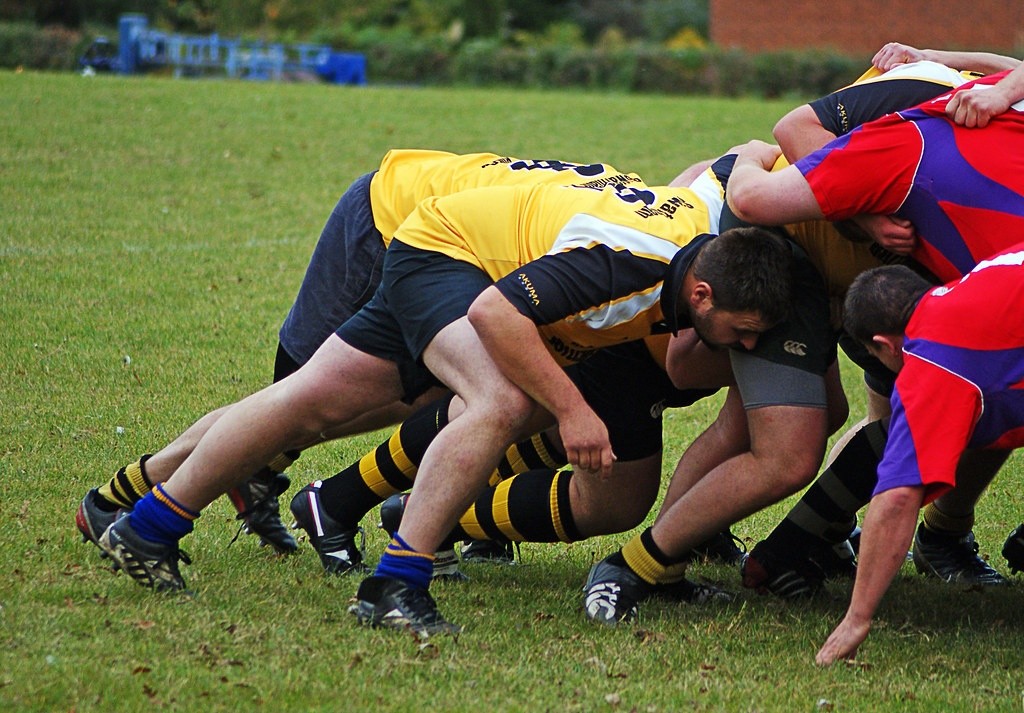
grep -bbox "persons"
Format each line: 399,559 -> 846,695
70,42 -> 1023,666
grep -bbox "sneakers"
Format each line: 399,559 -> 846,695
98,513 -> 192,593
826,540 -> 861,578
228,464 -> 300,556
913,521 -> 1008,587
290,480 -> 370,580
583,550 -> 657,629
688,525 -> 748,567
847,524 -> 915,560
462,537 -> 522,569
348,574 -> 461,643
1001,524 -> 1024,571
378,490 -> 460,581
76,490 -> 131,547
741,537 -> 831,612
659,575 -> 744,615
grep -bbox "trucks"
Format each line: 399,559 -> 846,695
74,10 -> 369,88
